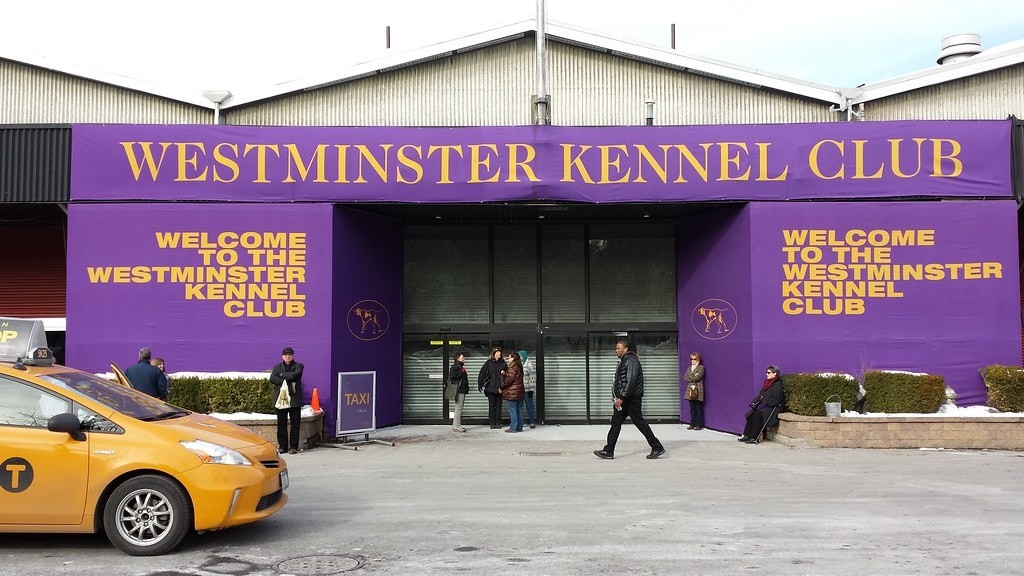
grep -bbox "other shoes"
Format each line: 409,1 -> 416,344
505,429 -> 512,432
694,425 -> 703,430
452,427 -> 466,433
530,423 -> 536,429
687,426 -> 694,429
738,436 -> 751,442
490,425 -> 497,429
745,438 -> 759,444
278,448 -> 288,454
289,448 -> 297,454
497,424 -> 501,429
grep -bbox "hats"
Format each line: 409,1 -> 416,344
282,347 -> 294,355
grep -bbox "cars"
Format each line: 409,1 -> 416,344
0,360 -> 290,556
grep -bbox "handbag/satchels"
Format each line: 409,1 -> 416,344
750,395 -> 763,410
275,379 -> 291,409
689,380 -> 697,400
482,360 -> 490,386
445,383 -> 460,400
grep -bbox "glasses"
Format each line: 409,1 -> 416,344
691,358 -> 697,360
766,372 -> 773,374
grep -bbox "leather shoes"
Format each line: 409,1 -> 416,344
646,448 -> 665,459
593,449 -> 614,459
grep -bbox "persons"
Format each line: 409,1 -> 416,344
683,351 -> 705,430
449,353 -> 470,431
498,352 -> 525,432
150,358 -> 173,394
270,346 -> 304,454
518,350 -> 536,429
737,365 -> 784,444
478,348 -> 509,429
594,339 -> 665,459
125,347 -> 167,400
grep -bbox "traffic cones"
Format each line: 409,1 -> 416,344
311,387 -> 324,414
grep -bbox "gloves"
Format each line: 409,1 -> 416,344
462,368 -> 466,373
